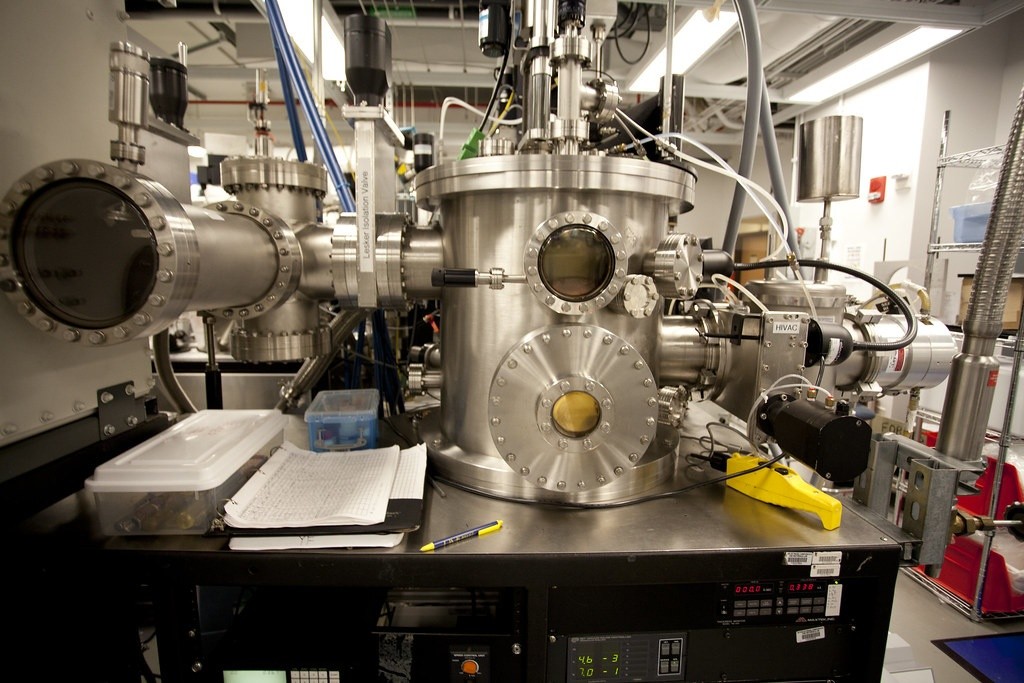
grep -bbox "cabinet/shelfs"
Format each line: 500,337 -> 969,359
894,109 -> 1024,621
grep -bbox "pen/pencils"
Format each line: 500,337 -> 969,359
419,519 -> 504,551
425,469 -> 446,498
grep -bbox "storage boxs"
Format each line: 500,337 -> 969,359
955,455 -> 1024,524
918,535 -> 1013,613
83,409 -> 290,537
951,201 -> 994,243
957,272 -> 1024,337
303,388 -> 379,453
